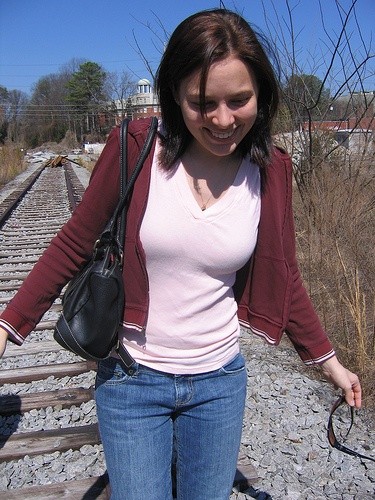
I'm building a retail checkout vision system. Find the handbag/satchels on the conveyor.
[52,115,159,367]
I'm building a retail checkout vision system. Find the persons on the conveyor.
[0,8,363,500]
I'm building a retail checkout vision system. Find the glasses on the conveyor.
[327,395,375,462]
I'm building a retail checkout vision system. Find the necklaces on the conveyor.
[187,144,233,212]
